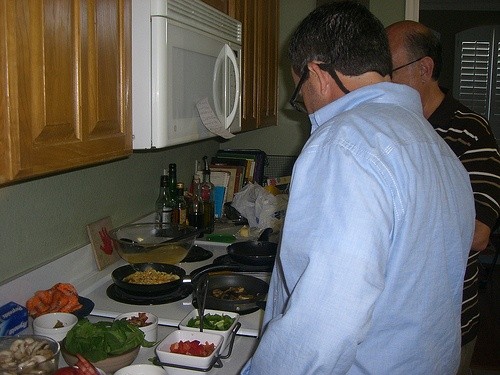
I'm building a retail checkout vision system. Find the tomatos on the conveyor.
[169,340,208,357]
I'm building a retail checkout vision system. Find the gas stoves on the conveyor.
[75,242,272,337]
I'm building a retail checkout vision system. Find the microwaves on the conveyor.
[131,0,243,151]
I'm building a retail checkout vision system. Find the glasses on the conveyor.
[288,62,329,114]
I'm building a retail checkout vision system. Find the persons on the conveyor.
[385,20,500,375]
[239,2,476,375]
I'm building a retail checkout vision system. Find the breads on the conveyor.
[240,226,250,237]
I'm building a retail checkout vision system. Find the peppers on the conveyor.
[187,313,234,331]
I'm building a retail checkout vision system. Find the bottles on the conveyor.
[154,162,214,238]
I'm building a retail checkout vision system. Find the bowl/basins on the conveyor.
[70,296,95,319]
[0,334,61,375]
[156,328,224,375]
[32,312,79,342]
[61,336,140,375]
[108,222,197,265]
[178,308,240,355]
[114,312,158,343]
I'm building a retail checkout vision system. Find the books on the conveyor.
[197,148,265,221]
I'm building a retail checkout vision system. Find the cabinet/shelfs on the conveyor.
[0,0,133,188]
[235,0,278,131]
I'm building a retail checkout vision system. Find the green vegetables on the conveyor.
[62,317,158,362]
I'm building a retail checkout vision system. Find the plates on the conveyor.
[113,364,206,375]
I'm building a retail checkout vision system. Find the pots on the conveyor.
[193,274,269,313]
[111,262,192,297]
[227,227,278,265]
